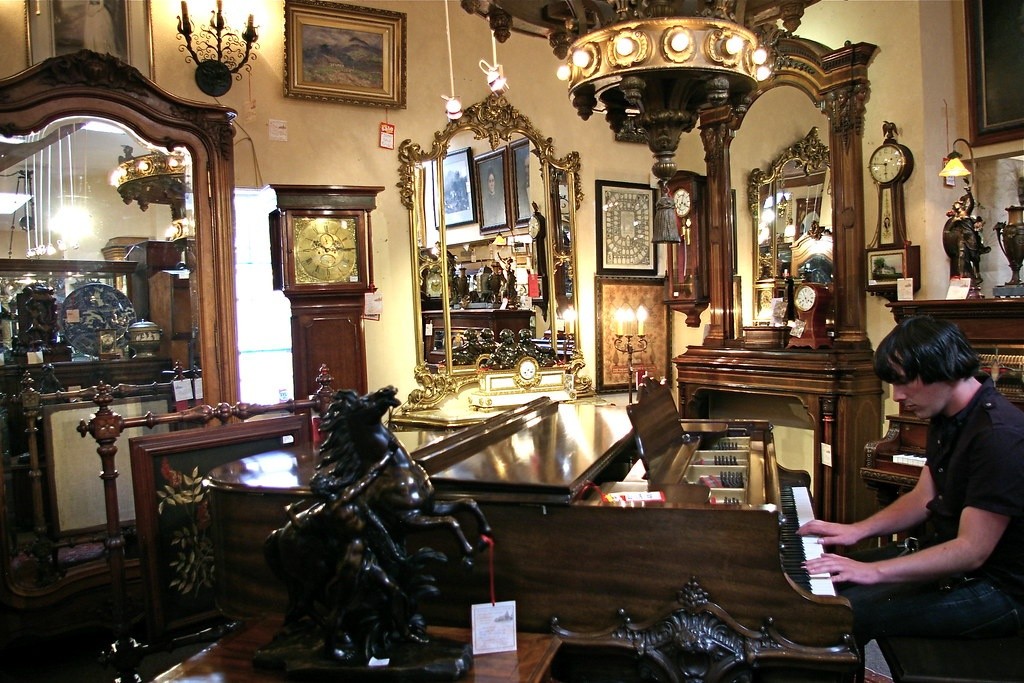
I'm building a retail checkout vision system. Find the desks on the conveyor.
[149,613,562,683]
[423,307,538,364]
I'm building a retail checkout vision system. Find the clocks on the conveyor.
[657,170,711,328]
[785,282,833,350]
[260,184,384,447]
[753,285,777,324]
[864,120,921,302]
[528,201,549,323]
[419,244,456,313]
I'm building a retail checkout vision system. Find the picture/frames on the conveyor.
[23,0,157,86]
[432,146,478,230]
[594,274,673,395]
[509,138,544,229]
[474,146,513,237]
[283,0,407,110]
[964,0,1024,149]
[595,179,658,277]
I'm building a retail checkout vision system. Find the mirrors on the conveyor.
[747,126,837,329]
[697,27,877,350]
[0,48,240,610]
[396,89,592,395]
[788,219,834,288]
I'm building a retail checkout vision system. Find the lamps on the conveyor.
[176,0,260,98]
[489,229,519,311]
[444,0,827,244]
[109,140,194,245]
[938,139,991,299]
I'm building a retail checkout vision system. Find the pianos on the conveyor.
[202,374,863,683]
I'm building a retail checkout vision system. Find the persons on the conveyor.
[488,328,517,370]
[517,328,561,367]
[482,168,503,224]
[320,533,405,662]
[464,329,498,355]
[283,436,407,568]
[793,313,1024,680]
[496,251,516,298]
[948,176,982,281]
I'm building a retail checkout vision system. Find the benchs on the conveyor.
[876,630,1023,683]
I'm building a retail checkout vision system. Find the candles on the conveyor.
[614,304,647,337]
